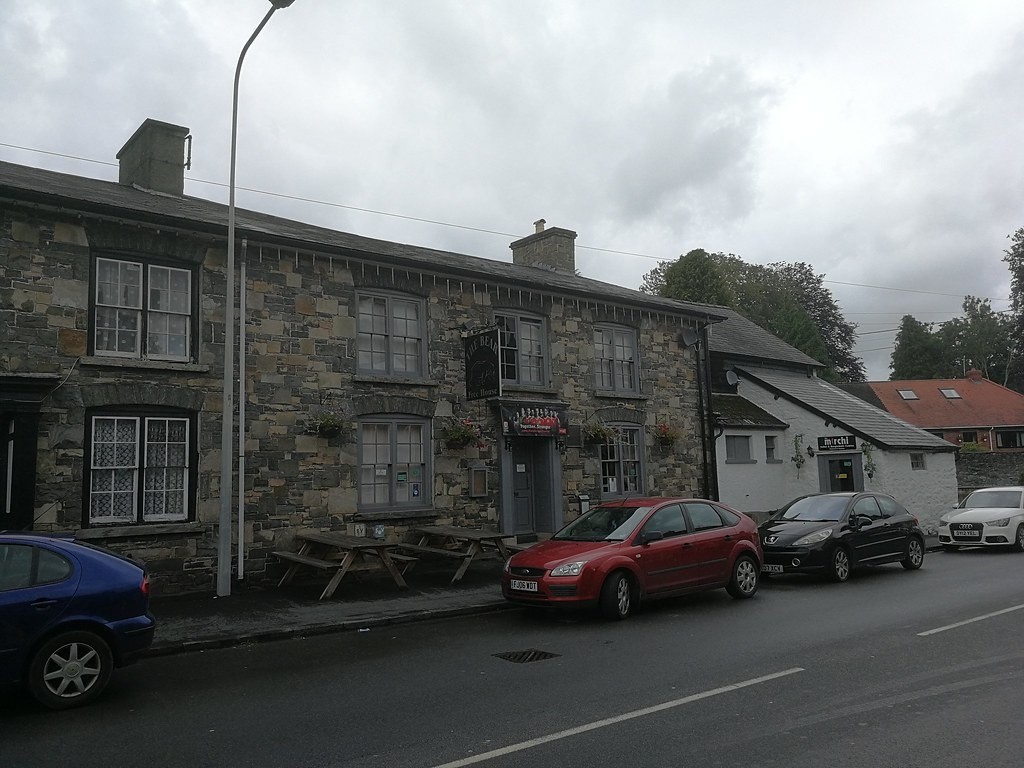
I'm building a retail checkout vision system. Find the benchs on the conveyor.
[271,549,344,572]
[458,538,529,553]
[344,546,418,565]
[398,542,472,560]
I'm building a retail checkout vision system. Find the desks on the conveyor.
[295,532,409,600]
[404,524,513,583]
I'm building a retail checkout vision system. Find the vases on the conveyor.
[446,435,473,450]
[658,436,674,448]
[590,434,610,446]
[317,422,346,438]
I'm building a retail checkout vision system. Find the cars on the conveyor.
[500,497,762,624]
[756,491,924,582]
[938,486,1023,551]
[1,529,152,717]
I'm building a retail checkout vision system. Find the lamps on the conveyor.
[806,446,815,458]
[503,435,512,451]
[556,433,567,449]
[860,443,868,455]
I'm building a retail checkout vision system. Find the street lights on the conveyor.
[215,1,295,596]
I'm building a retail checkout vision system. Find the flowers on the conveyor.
[434,416,493,453]
[651,424,682,441]
[578,419,626,445]
[298,391,359,426]
